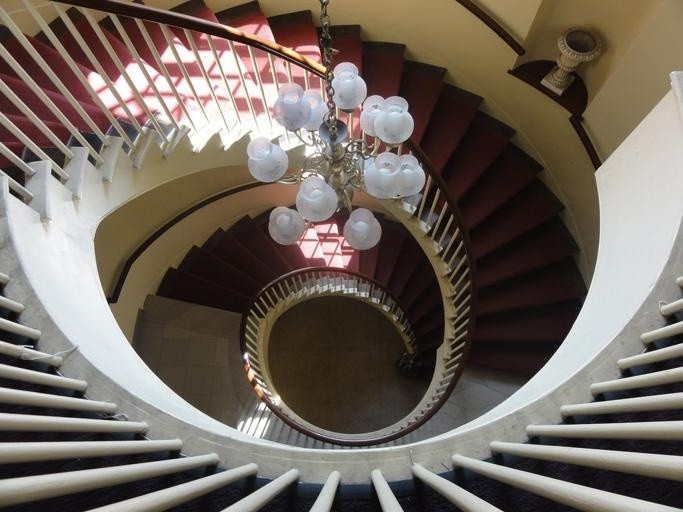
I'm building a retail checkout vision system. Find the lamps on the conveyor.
[241,1,428,253]
